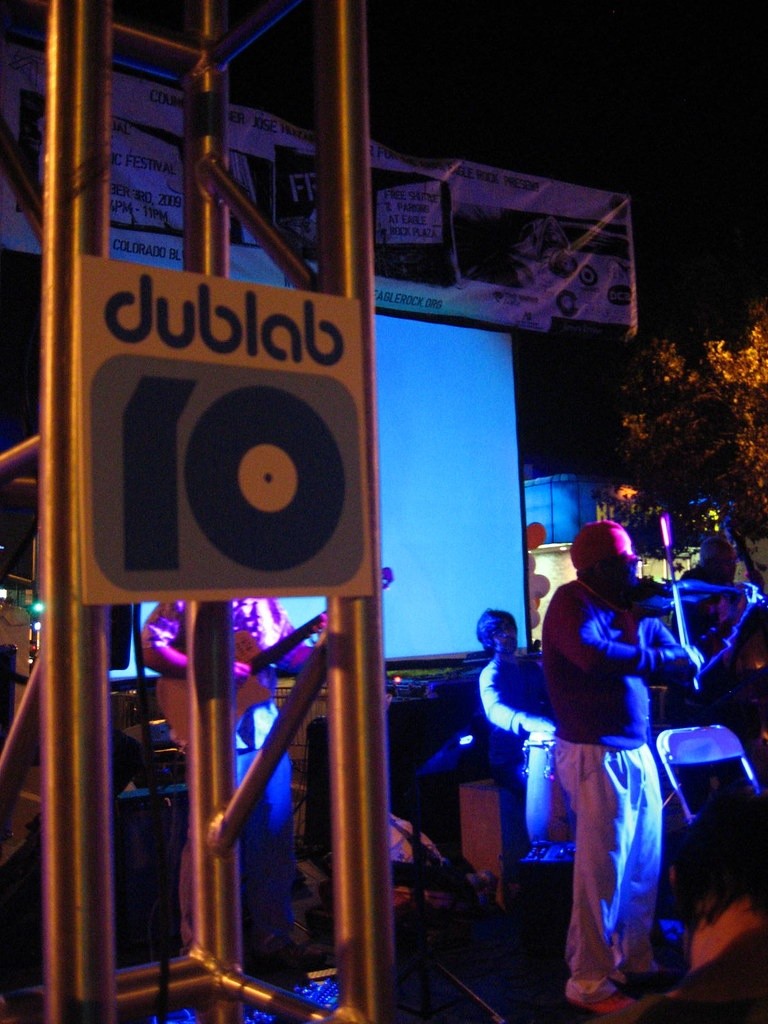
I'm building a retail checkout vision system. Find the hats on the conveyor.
[569,519,642,575]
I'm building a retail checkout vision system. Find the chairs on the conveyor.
[657,723,763,826]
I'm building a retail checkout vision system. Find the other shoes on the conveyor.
[610,960,675,992]
[564,977,638,1016]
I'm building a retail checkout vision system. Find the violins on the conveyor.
[628,576,768,615]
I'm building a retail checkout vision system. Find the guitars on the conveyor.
[154,566,395,744]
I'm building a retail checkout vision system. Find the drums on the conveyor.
[519,727,576,850]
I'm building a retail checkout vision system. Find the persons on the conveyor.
[541,520,768,1014]
[138,596,331,968]
[580,786,767,1024]
[473,608,549,795]
